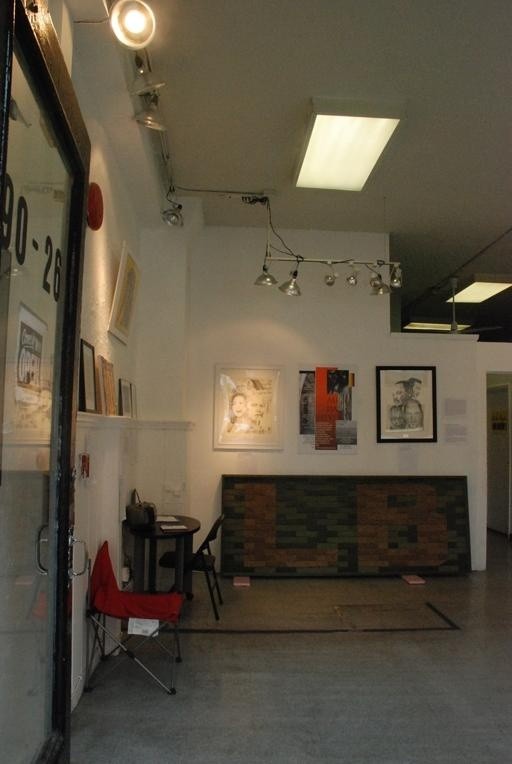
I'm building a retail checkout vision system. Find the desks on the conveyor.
[121,514,200,601]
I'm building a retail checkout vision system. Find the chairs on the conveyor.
[158,511,226,621]
[83,539,187,695]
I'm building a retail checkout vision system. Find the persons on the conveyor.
[224,378,268,433]
[390,377,424,430]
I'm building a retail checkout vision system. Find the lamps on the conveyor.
[295,112,400,192]
[254,245,304,296]
[322,259,403,296]
[161,186,183,230]
[111,2,169,138]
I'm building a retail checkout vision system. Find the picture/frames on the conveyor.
[375,364,438,443]
[79,237,139,420]
[213,363,284,452]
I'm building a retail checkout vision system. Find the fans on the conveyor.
[445,276,504,334]
